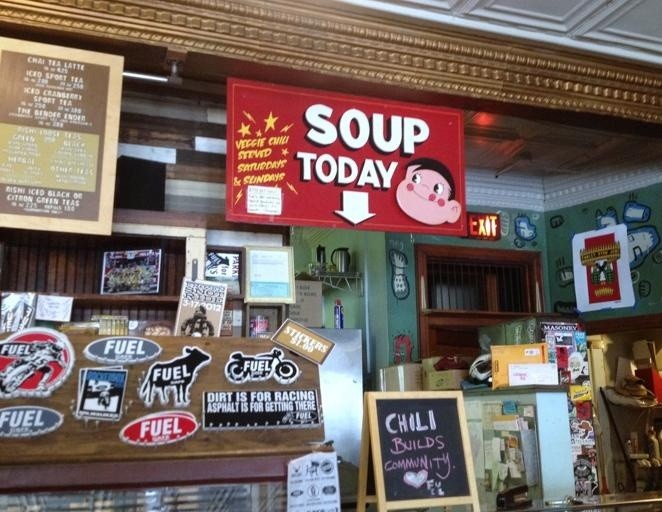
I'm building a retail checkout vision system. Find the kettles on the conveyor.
[330,246,351,273]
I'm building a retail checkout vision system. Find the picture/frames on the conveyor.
[244,244,296,304]
[204,245,246,300]
[99,245,163,295]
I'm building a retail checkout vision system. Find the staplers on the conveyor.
[496,485,533,512]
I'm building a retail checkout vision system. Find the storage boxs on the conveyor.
[421,355,473,392]
[428,369,468,392]
[478,317,583,373]
[378,363,423,393]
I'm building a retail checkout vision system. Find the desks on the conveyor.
[495,492,662,512]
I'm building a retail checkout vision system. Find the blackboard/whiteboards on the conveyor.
[367,390,477,510]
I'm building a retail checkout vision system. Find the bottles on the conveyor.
[333,299,344,329]
[647,426,661,458]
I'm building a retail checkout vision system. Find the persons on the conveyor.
[103,261,153,293]
[396,157,462,227]
[0,340,66,392]
